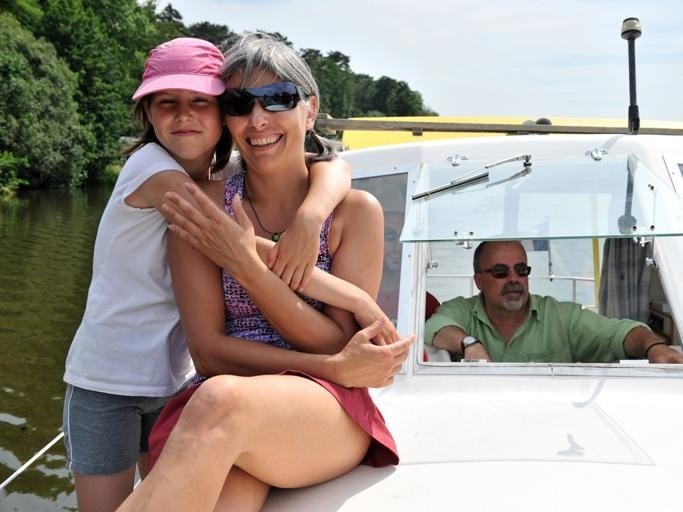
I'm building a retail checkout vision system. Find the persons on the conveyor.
[376,210,456,362]
[112,34,417,512]
[425,241,683,364]
[61,37,402,512]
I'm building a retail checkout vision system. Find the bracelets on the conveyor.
[644,342,669,358]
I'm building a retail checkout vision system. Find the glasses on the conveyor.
[476,263,531,278]
[221,81,308,116]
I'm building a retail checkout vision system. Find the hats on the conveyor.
[131,37,227,101]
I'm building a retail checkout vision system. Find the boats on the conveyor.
[0,16,682,510]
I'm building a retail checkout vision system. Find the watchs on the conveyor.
[461,336,484,354]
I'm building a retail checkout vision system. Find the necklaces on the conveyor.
[244,173,287,242]
